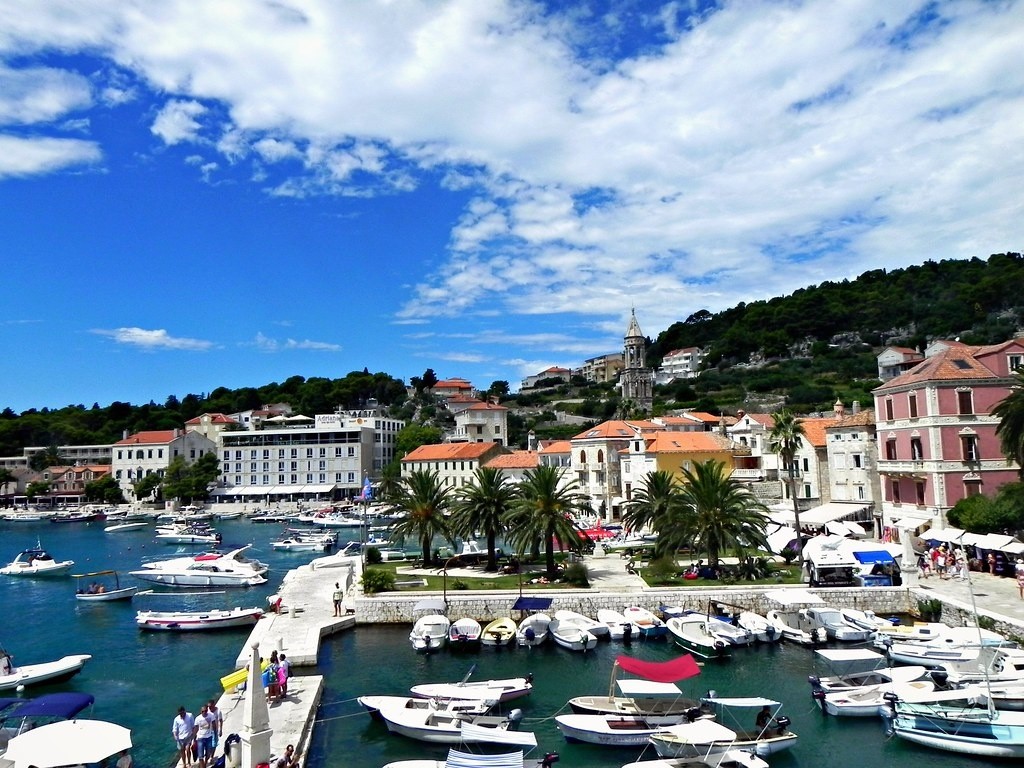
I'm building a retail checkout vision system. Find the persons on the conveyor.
[1015,559,1024,599]
[254,507,261,512]
[28,554,34,567]
[988,552,995,574]
[917,545,966,579]
[277,744,299,768]
[89,582,104,593]
[677,559,718,580]
[333,582,343,616]
[266,596,282,614]
[173,700,223,768]
[268,650,292,703]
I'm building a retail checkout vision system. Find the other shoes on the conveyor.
[333,614,336,617]
[280,695,286,698]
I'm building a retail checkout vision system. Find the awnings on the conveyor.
[893,516,929,532]
[300,483,336,493]
[269,484,304,494]
[210,486,246,494]
[786,502,873,534]
[239,485,274,494]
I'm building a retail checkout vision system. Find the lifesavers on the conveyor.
[799,613,804,619]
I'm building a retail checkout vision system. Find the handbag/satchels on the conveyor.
[288,666,293,676]
[191,740,198,762]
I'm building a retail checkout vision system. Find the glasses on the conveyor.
[202,710,208,712]
[289,750,294,752]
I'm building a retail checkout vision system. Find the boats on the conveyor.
[647,696,799,758]
[621,747,770,768]
[409,588,1024,710]
[0,500,659,587]
[380,706,523,744]
[808,666,926,695]
[410,662,534,706]
[380,748,560,768]
[880,533,1024,759]
[812,679,935,717]
[134,606,265,630]
[75,586,137,602]
[356,694,498,723]
[0,535,74,577]
[568,653,703,716]
[554,710,717,747]
[0,639,92,690]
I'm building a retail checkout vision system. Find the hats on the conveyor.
[964,549,967,551]
[266,595,269,602]
[924,551,929,554]
[1017,559,1023,563]
[941,543,946,546]
